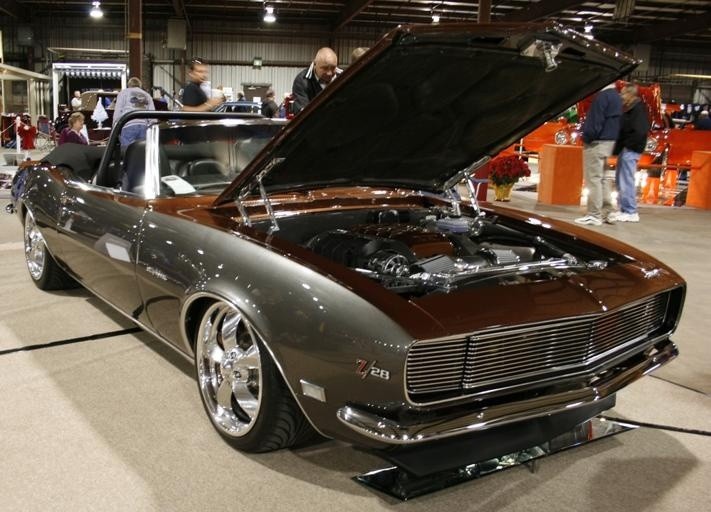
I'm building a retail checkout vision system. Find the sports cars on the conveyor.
[9,18,687,483]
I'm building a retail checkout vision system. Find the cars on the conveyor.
[52,91,169,139]
[554,77,676,165]
[279,92,294,119]
[213,101,289,120]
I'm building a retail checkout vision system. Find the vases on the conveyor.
[492,184,515,202]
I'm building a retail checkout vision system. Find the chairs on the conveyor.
[122,138,169,194]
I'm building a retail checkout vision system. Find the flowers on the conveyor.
[489,155,532,186]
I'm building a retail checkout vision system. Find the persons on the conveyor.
[60,112,107,146]
[574,82,622,228]
[112,77,159,149]
[181,46,371,127]
[71,90,83,111]
[611,85,648,222]
[693,110,711,130]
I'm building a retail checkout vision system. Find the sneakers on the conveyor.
[575,204,641,226]
